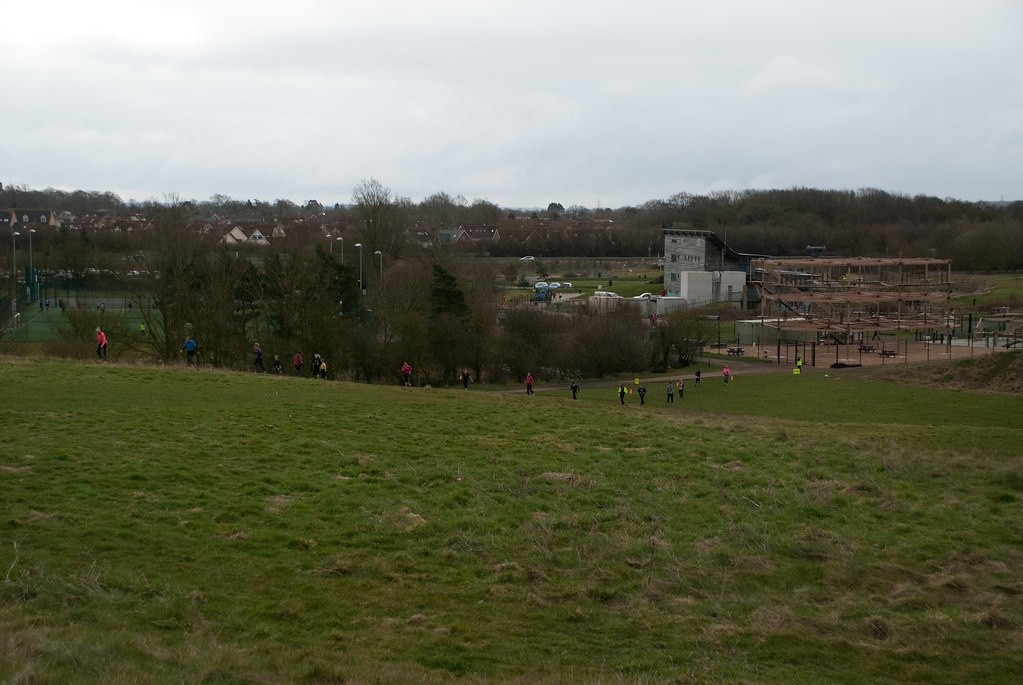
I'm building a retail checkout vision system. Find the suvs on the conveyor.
[519,256,536,264]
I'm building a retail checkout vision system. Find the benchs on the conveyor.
[858,348,867,350]
[732,350,744,353]
[878,352,889,355]
[727,350,737,352]
[887,353,897,356]
[867,349,876,350]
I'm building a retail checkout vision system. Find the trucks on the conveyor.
[594,291,621,298]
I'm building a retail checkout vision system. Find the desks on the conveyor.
[727,347,744,355]
[878,349,896,358]
[859,345,875,353]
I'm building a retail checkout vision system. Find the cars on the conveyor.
[549,282,561,289]
[562,283,573,289]
[532,281,548,290]
[0,267,162,286]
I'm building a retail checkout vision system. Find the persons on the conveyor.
[617,385,628,405]
[678,379,684,398]
[58,296,65,317]
[294,351,303,377]
[665,381,674,403]
[695,369,700,386]
[570,381,580,400]
[39,300,43,315]
[45,301,50,312]
[312,354,322,380]
[524,373,535,396]
[721,365,731,385]
[401,361,411,387]
[95,327,107,363]
[459,368,474,390]
[319,359,328,381]
[272,355,281,374]
[179,336,200,368]
[139,322,146,335]
[797,355,802,372]
[638,385,646,405]
[95,302,105,313]
[253,342,267,374]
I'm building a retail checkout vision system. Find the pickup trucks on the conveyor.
[633,292,662,299]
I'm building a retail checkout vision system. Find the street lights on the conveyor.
[374,250,384,289]
[354,243,362,291]
[336,237,344,264]
[28,229,38,281]
[326,234,332,254]
[11,231,21,279]
